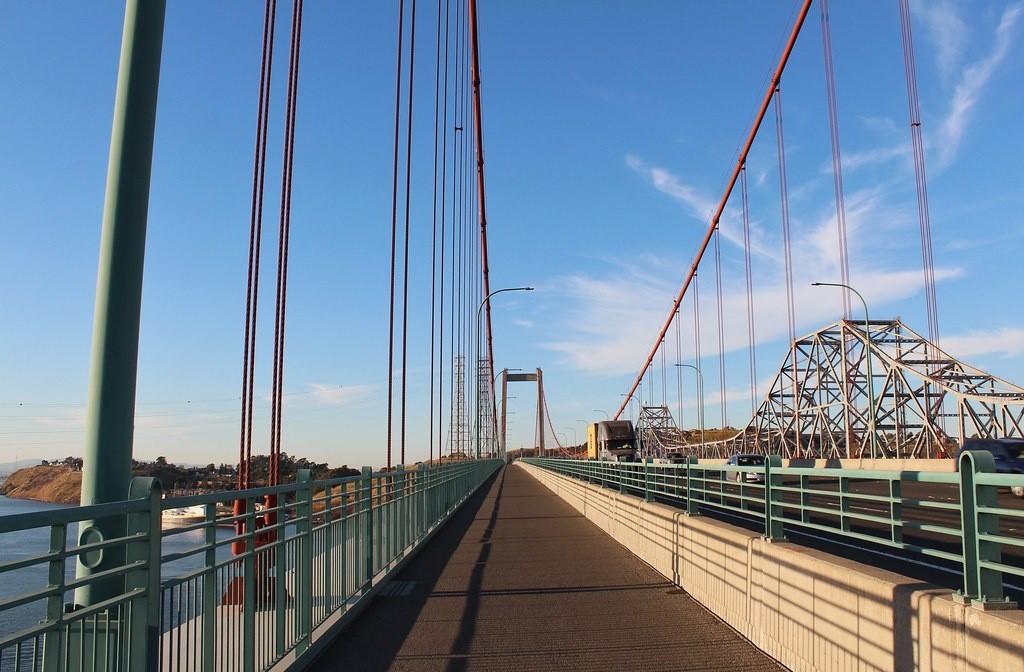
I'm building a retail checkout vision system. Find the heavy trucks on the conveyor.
[585,420,638,463]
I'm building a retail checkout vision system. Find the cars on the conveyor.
[724,454,765,484]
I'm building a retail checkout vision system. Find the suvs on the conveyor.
[955,438,1024,498]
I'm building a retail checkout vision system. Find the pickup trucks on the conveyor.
[659,453,684,469]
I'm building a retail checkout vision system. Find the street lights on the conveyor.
[811,282,878,458]
[577,419,589,426]
[673,363,705,459]
[475,286,535,460]
[547,426,577,460]
[593,409,609,421]
[620,393,642,411]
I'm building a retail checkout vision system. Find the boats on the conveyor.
[159,504,269,530]
